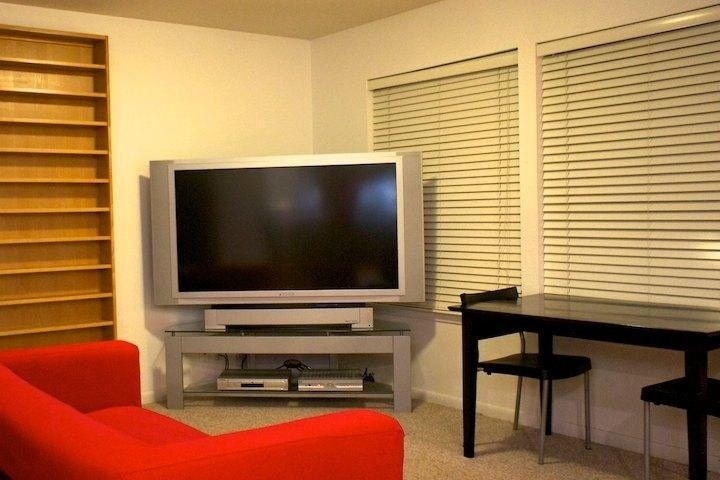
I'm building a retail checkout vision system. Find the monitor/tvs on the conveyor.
[149,150,425,306]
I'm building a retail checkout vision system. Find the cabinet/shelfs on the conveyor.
[0,23,116,348]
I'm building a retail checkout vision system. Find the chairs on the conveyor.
[460,286,592,465]
[640,376,720,480]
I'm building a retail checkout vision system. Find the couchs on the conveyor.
[0,341,404,480]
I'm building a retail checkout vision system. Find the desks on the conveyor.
[163,319,412,413]
[447,292,720,480]
